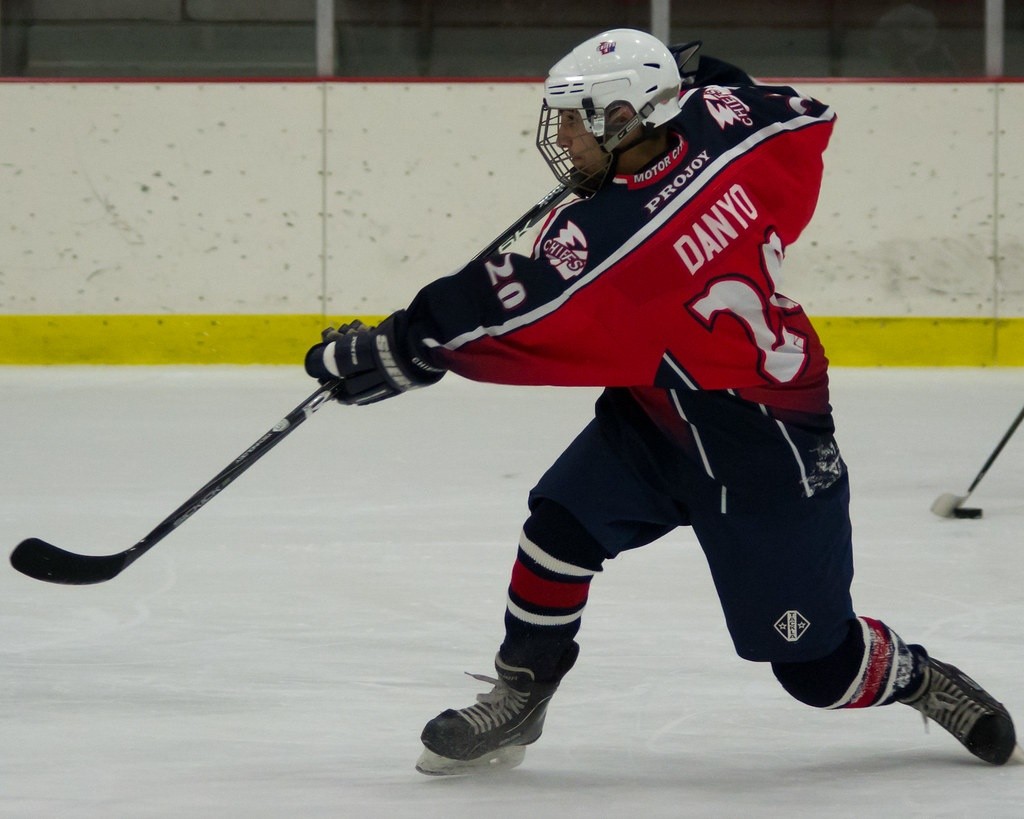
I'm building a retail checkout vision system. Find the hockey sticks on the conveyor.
[8,166,581,588]
[928,408,1024,520]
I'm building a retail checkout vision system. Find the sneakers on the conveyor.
[415,641,580,776]
[900,645,1024,766]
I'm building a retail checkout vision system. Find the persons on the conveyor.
[305,27,1023,775]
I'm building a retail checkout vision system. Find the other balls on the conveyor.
[952,507,983,520]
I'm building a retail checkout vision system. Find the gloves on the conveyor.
[305,308,447,407]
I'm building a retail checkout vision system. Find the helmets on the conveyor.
[536,29,683,199]
